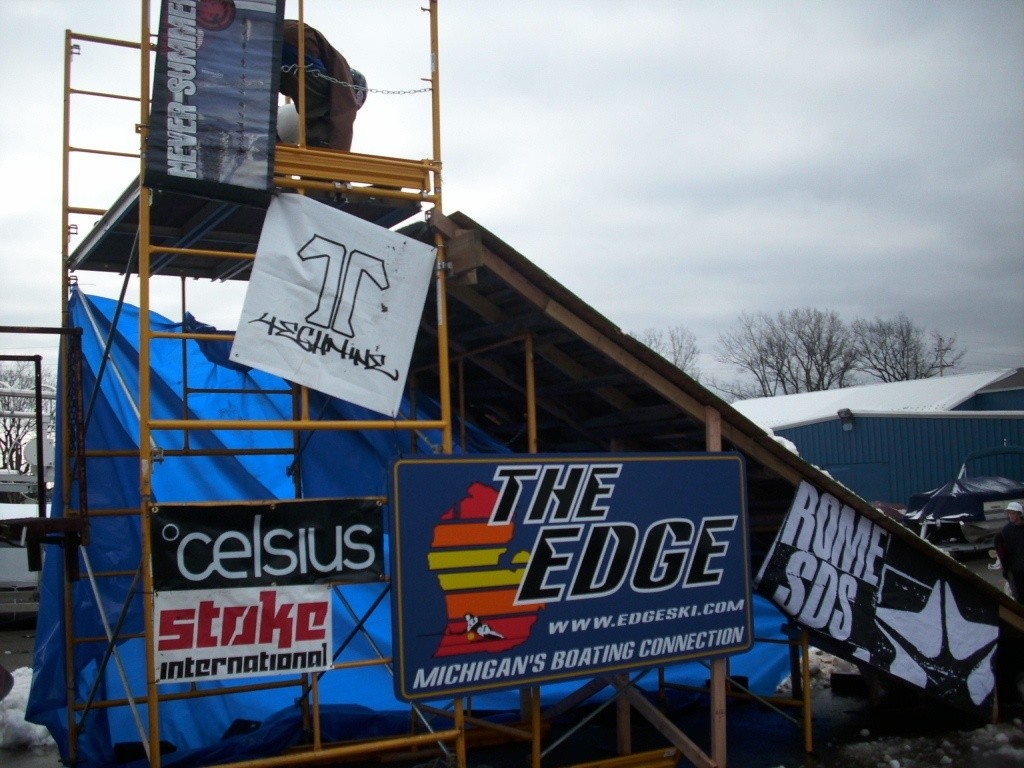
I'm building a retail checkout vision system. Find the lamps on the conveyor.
[837,408,855,431]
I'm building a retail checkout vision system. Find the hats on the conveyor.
[1005,502,1023,513]
[350,68,369,111]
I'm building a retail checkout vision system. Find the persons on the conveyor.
[996,502,1024,609]
[280,19,358,196]
